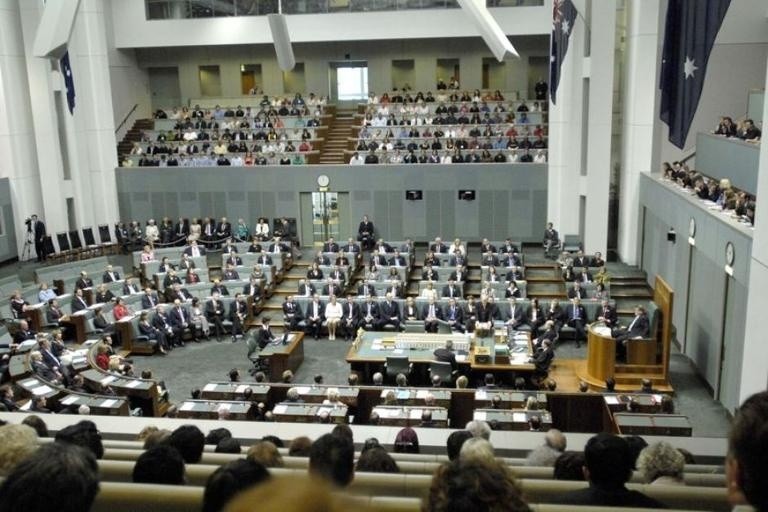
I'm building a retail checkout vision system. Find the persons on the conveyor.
[119,76,549,169]
[663,115,761,224]
[0,215,767,511]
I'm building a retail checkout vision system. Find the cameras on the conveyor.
[24,217,32,225]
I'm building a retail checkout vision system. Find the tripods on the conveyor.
[20,225,35,262]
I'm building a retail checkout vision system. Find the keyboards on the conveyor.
[272,340,282,345]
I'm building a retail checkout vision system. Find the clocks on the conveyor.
[316,174,330,187]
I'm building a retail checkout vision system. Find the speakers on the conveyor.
[668,230,675,243]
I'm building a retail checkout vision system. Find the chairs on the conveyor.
[543,230,560,250]
[563,235,582,253]
[45,217,300,266]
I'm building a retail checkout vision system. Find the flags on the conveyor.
[550,1,577,104]
[660,1,730,150]
[60,51,75,114]
